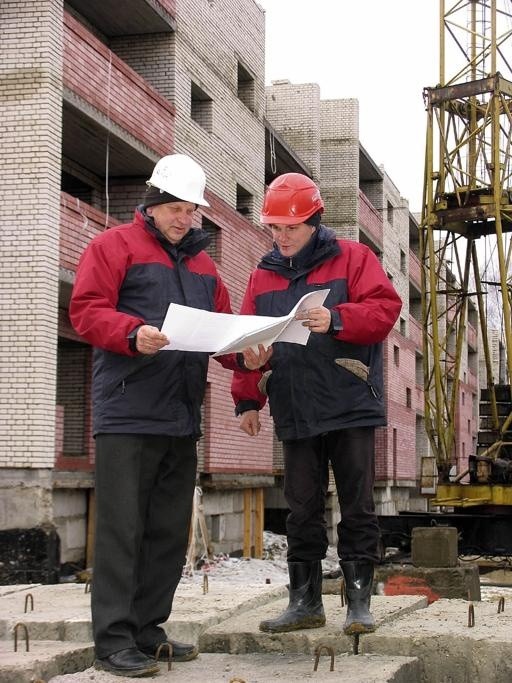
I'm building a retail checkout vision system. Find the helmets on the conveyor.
[258,172,324,225]
[145,154,210,208]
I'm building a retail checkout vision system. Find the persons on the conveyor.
[227,168,404,640]
[66,151,277,679]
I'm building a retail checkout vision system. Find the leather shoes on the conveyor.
[93,646,160,677]
[141,634,199,662]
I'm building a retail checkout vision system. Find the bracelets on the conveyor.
[243,358,251,370]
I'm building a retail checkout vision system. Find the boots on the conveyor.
[338,559,377,635]
[258,560,326,632]
